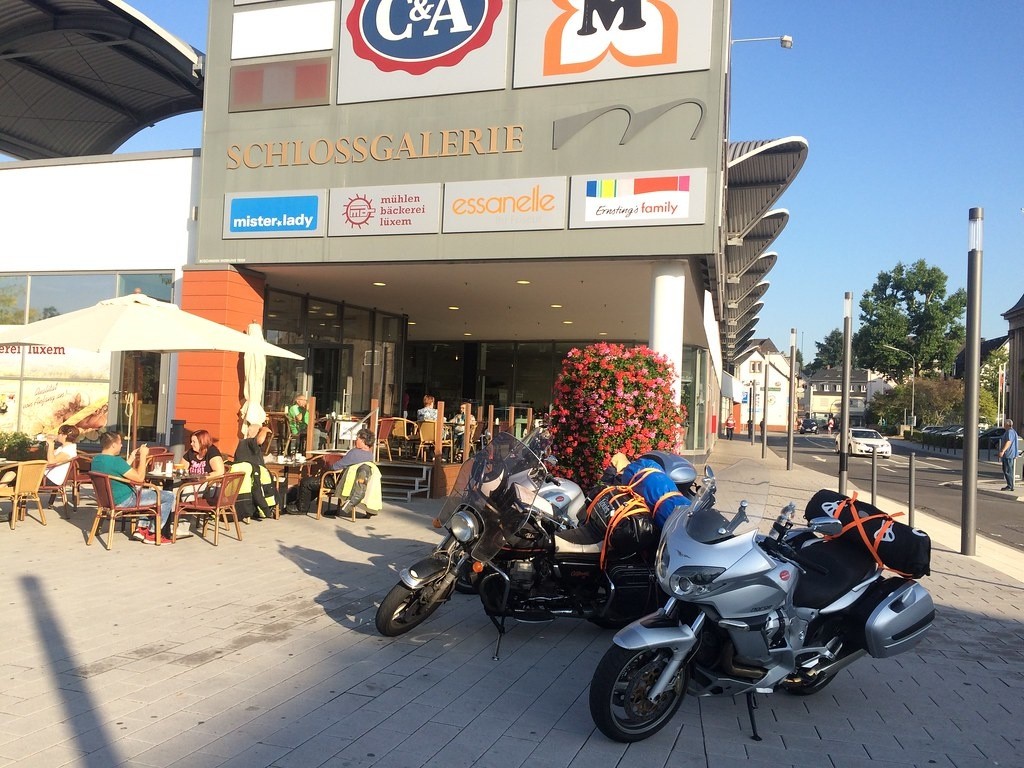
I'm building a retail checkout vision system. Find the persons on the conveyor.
[0,424,80,487]
[828,418,834,428]
[417,395,449,461]
[234,425,272,467]
[760,418,764,435]
[170,430,224,535]
[449,402,475,461]
[725,413,736,440]
[287,395,321,450]
[284,429,375,515]
[90,432,174,545]
[998,419,1018,490]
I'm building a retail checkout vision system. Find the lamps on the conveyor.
[731,34,794,49]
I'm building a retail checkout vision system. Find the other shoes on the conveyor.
[0,512,20,523]
[1001,486,1014,492]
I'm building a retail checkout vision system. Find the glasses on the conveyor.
[58,432,65,435]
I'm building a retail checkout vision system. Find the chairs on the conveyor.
[0,447,279,550]
[298,454,382,522]
[265,411,489,465]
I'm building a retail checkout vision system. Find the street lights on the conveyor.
[883,345,915,436]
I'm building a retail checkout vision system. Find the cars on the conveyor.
[800,419,819,435]
[978,427,1023,449]
[834,429,892,459]
[921,425,964,439]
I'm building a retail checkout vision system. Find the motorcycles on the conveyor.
[376,423,701,661]
[588,457,935,743]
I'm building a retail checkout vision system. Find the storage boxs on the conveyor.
[855,577,935,659]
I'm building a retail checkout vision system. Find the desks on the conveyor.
[265,461,317,515]
[145,470,210,540]
[318,417,368,450]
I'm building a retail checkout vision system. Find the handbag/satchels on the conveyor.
[622,459,693,526]
[803,488,933,578]
[203,480,221,507]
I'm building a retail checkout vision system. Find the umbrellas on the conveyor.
[0,287,306,466]
[239,321,267,439]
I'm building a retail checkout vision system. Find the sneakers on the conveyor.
[143,532,172,545]
[287,503,307,515]
[133,527,151,540]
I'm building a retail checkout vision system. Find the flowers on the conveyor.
[523,342,690,496]
